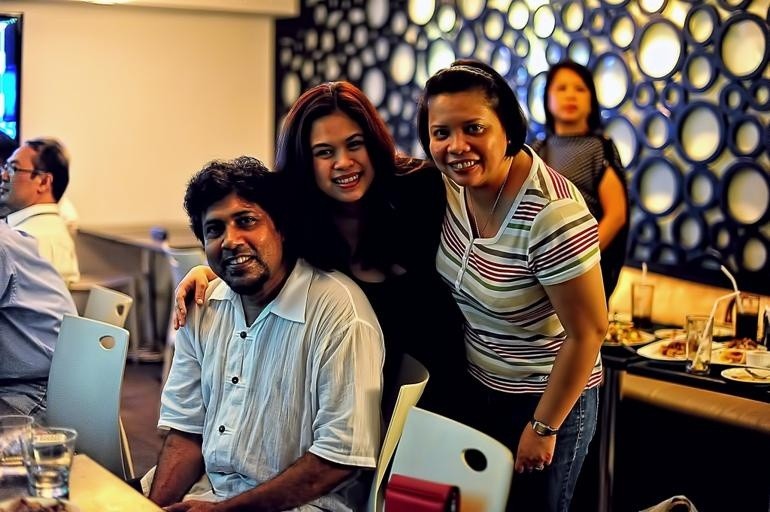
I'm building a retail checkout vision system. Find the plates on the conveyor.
[1,496,81,512]
[653,328,686,339]
[600,329,657,347]
[721,367,770,384]
[635,339,690,361]
[705,348,770,369]
[719,341,767,350]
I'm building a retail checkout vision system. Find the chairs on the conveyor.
[382,406,514,512]
[367,352,431,512]
[75,221,205,364]
[161,239,204,390]
[70,276,139,363]
[82,285,134,479]
[46,312,131,483]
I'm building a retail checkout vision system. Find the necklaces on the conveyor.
[465,166,509,239]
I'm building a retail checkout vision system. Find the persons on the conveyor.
[415,59,610,512]
[0,217,80,431]
[1,137,81,296]
[173,80,460,512]
[123,155,387,512]
[524,60,630,311]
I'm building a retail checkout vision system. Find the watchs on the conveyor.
[530,415,559,437]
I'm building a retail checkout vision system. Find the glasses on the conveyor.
[1,163,33,176]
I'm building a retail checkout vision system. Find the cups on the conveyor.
[685,313,714,375]
[631,283,654,330]
[19,426,79,501]
[744,350,769,367]
[0,413,35,467]
[735,293,760,343]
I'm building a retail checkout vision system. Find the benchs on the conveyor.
[608,259,770,432]
